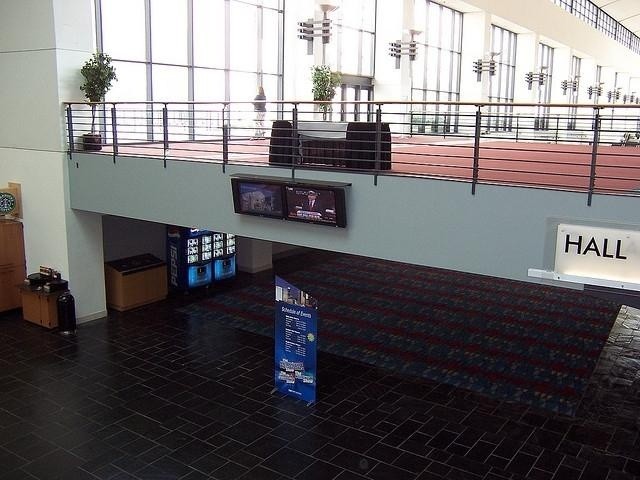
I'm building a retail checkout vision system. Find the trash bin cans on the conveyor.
[56,290,76,336]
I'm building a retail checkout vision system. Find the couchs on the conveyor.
[268,120,392,170]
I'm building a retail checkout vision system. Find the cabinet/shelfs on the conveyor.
[104,253,168,313]
[0,219,27,312]
[15,288,76,330]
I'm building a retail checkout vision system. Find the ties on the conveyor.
[309,200,313,211]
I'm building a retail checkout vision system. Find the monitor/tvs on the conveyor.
[231,178,347,228]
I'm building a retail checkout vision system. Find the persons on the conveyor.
[251,87,268,139]
[302,190,323,215]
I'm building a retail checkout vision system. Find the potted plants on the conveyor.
[79,46,119,150]
